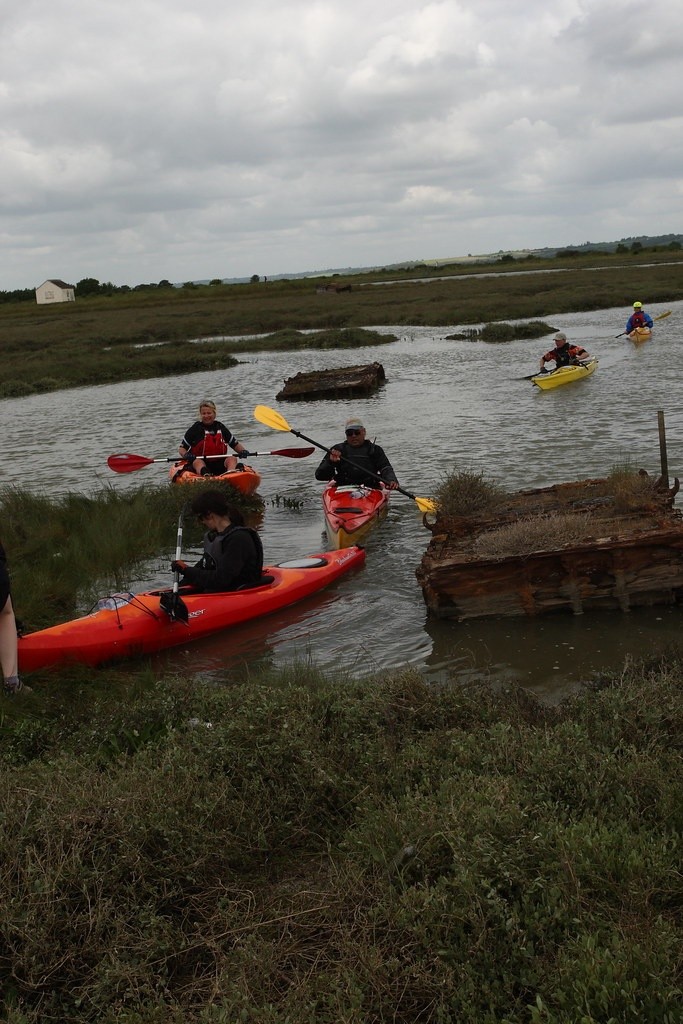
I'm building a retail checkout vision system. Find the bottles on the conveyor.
[98,593,124,610]
[107,593,135,611]
[538,374,549,377]
[351,490,368,499]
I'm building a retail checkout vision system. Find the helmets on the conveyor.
[633,302,642,311]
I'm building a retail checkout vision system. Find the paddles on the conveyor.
[521,361,575,379]
[254,405,443,512]
[159,500,190,627]
[108,447,315,473]
[615,311,671,338]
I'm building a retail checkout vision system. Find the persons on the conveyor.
[171,489,255,594]
[540,332,589,374]
[624,301,653,335]
[179,400,248,477]
[0,542,35,697]
[315,417,399,491]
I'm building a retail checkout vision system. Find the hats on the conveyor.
[345,418,363,432]
[553,333,566,340]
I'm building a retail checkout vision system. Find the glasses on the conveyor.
[199,512,213,522]
[346,430,361,436]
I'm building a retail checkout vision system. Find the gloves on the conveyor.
[171,560,187,574]
[239,450,249,459]
[569,359,579,365]
[540,367,548,374]
[184,453,196,462]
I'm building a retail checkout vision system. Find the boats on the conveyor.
[170,461,261,494]
[18,544,366,674]
[531,356,599,389]
[323,480,389,551]
[629,327,652,342]
